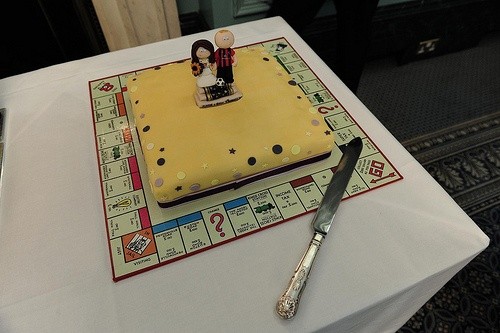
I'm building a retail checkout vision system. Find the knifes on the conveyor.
[276,135,366,320]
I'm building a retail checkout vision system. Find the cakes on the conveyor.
[126,30,335,210]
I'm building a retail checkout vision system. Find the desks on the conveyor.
[0,13,490,332]
